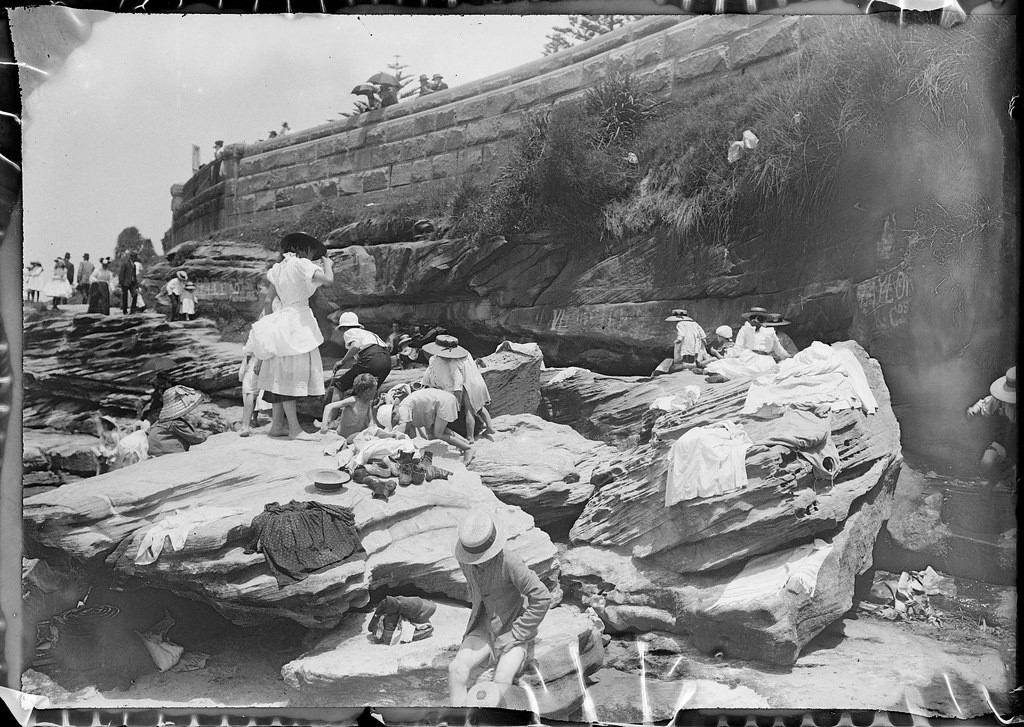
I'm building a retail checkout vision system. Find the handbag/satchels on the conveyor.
[367,594,436,645]
[136,293,146,308]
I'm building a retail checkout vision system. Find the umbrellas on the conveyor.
[351,84,377,95]
[366,73,404,88]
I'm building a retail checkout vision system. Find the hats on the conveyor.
[421,335,468,359]
[279,232,327,261]
[664,309,694,322]
[157,385,203,424]
[741,308,767,321]
[334,311,364,332]
[31,251,110,267]
[376,399,398,433]
[306,468,352,491]
[990,366,1016,404]
[755,312,791,326]
[185,281,195,290]
[715,324,734,339]
[451,510,508,564]
[398,334,411,347]
[177,270,188,281]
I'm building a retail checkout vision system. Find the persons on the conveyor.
[24,250,144,315]
[418,72,448,97]
[664,306,791,374]
[965,364,1016,491]
[268,121,290,138]
[367,84,399,108]
[314,310,496,466]
[237,230,335,442]
[146,385,208,456]
[165,269,200,321]
[447,515,551,708]
[89,256,113,316]
[212,139,225,158]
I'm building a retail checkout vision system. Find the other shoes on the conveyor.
[696,360,706,368]
[691,367,705,374]
[705,375,723,382]
[350,448,452,503]
